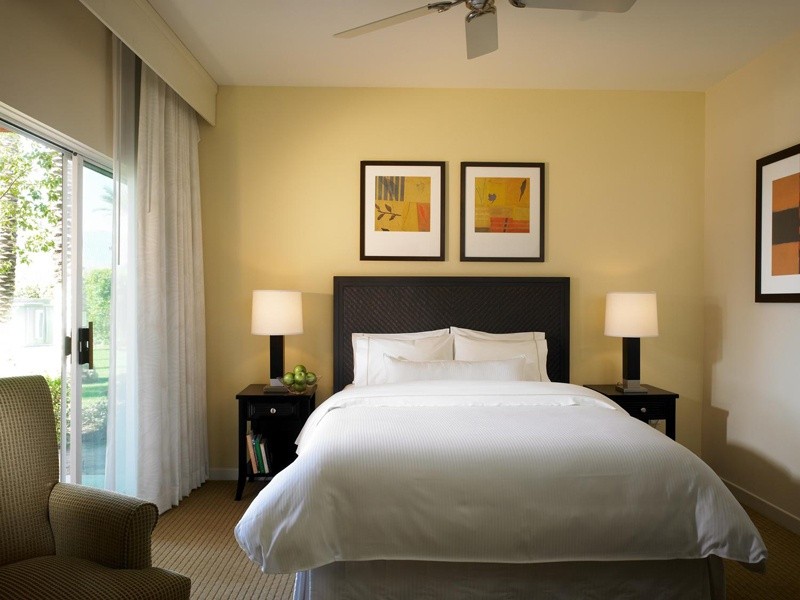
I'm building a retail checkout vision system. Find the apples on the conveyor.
[284,364,317,392]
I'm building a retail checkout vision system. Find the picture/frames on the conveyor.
[460,161,544,262]
[755,143,800,303]
[360,160,445,261]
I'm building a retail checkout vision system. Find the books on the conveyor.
[246,431,270,473]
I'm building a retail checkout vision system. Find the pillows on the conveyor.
[451,325,553,382]
[451,354,526,383]
[373,352,456,385]
[353,327,454,384]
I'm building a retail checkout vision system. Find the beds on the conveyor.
[233,276,769,600]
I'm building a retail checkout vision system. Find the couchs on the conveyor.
[0,374,191,600]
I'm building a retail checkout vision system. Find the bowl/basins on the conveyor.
[279,377,320,395]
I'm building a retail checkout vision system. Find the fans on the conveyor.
[334,0,637,59]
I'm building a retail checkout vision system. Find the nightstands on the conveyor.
[582,383,680,441]
[234,384,318,502]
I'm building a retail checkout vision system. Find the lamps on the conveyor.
[604,292,658,394]
[251,289,303,391]
